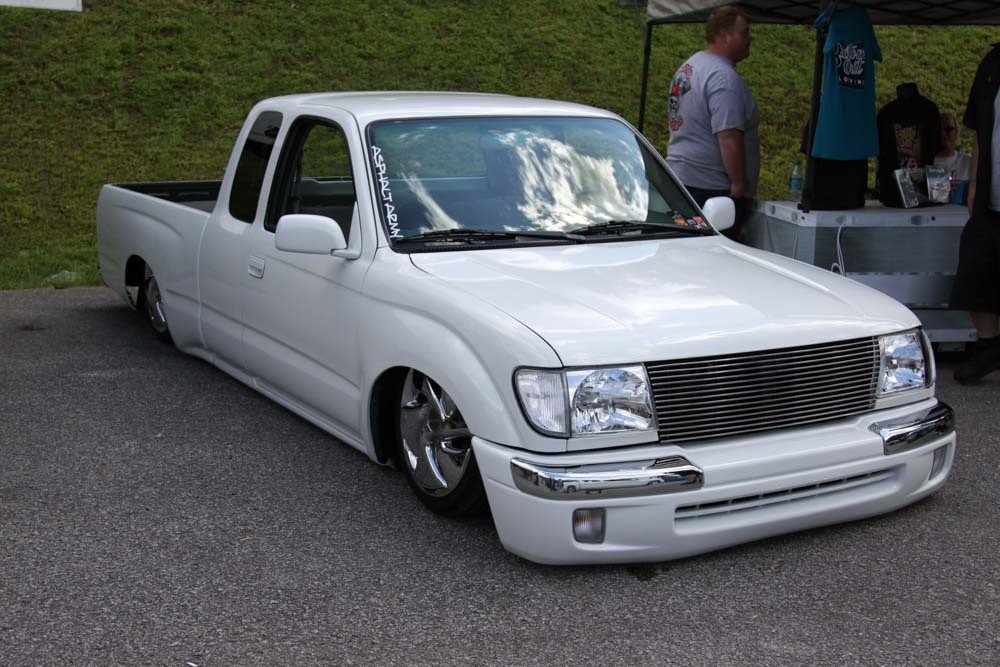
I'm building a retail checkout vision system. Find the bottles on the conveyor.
[788,159,803,202]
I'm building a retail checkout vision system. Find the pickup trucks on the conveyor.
[93,88,958,566]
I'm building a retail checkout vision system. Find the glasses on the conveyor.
[941,125,960,132]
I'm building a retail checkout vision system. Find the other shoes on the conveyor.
[954,336,1000,382]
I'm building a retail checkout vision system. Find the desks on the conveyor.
[749,200,977,353]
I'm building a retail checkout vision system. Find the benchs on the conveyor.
[295,178,543,232]
